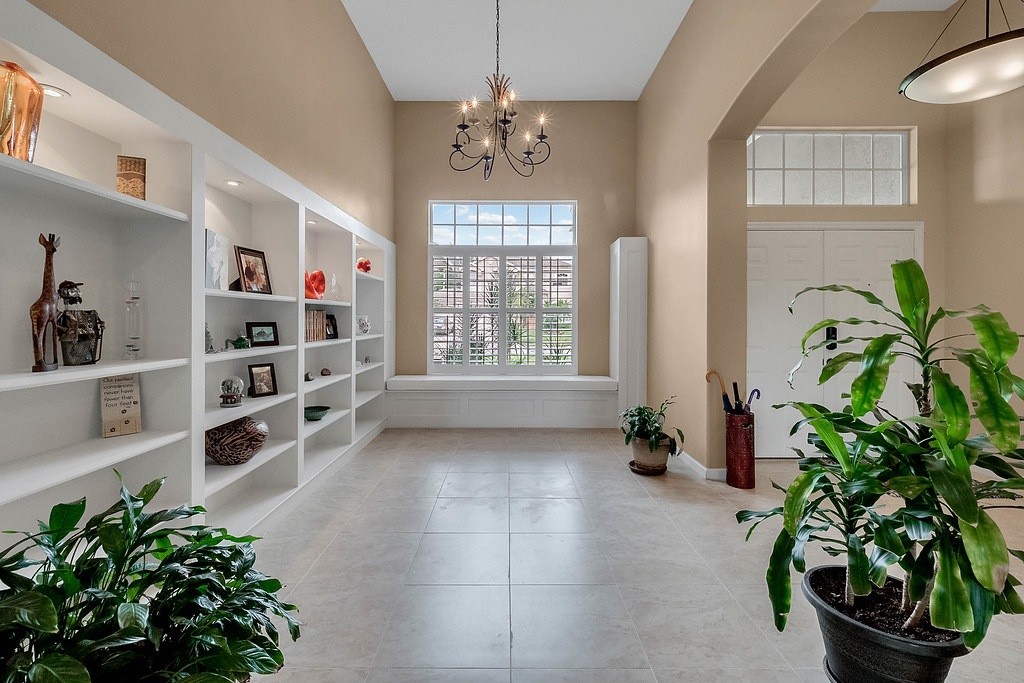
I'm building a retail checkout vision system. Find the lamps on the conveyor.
[449,0,551,182]
[897,0,1024,105]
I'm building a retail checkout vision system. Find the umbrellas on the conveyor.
[705,370,761,415]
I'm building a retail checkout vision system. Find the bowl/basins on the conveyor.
[303,406,332,421]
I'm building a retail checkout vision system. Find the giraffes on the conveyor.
[30,232,60,366]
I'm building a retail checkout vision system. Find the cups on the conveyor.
[0,60,44,163]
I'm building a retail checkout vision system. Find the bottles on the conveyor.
[126,281,145,360]
[356,315,372,336]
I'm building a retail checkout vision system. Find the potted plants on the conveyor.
[733,258,1024,683]
[618,394,685,477]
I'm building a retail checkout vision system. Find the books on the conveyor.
[305,310,326,342]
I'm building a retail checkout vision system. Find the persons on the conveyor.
[245,263,257,282]
[257,372,269,385]
[256,328,266,336]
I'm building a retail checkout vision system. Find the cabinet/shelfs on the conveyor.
[0,0,398,662]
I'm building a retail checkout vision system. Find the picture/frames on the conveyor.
[245,321,280,347]
[248,362,279,398]
[234,245,272,295]
[326,314,338,339]
